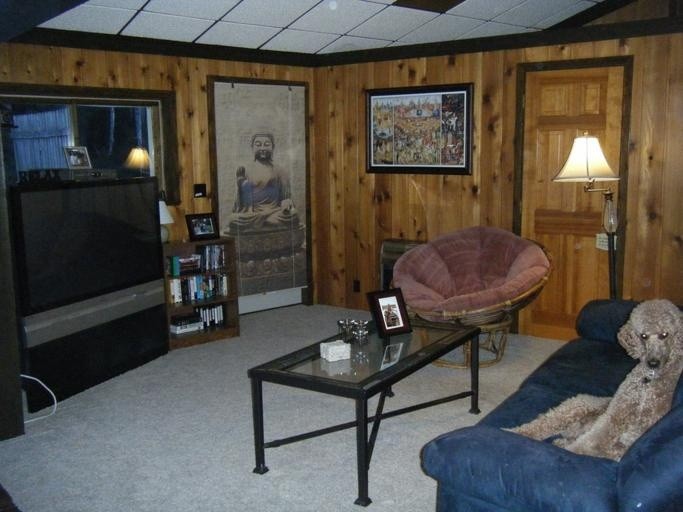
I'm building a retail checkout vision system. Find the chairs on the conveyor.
[392,226,552,367]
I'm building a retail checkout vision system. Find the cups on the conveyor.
[337,318,368,347]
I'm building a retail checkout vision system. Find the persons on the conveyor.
[384,301,399,328]
[383,343,399,365]
[227,128,298,236]
[372,137,464,166]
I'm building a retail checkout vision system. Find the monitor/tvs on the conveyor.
[7,177,169,348]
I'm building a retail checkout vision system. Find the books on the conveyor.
[165,240,230,335]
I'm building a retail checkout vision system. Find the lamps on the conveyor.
[124,147,152,177]
[551,131,621,301]
[159,200,175,243]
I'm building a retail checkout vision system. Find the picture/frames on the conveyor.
[206,73,314,291]
[63,146,93,170]
[186,214,217,239]
[365,287,412,338]
[365,82,474,176]
[373,335,413,374]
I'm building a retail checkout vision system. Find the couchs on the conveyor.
[419,298,683,512]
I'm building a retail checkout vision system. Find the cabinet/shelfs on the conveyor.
[164,239,241,347]
